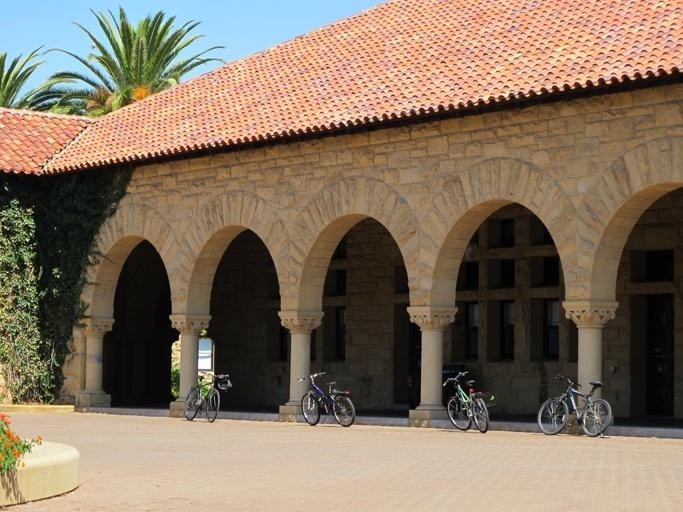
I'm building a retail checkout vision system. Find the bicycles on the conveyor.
[184,372,229,422]
[298,372,355,427]
[443,371,489,433]
[538,374,612,437]
